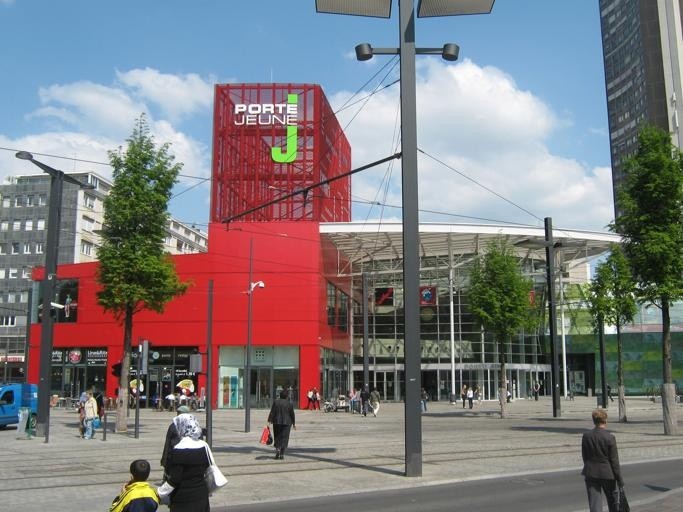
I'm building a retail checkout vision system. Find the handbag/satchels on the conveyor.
[620,488,630,511]
[93,419,101,428]
[259,428,273,446]
[204,464,228,493]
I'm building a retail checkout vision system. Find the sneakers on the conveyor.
[276,450,283,459]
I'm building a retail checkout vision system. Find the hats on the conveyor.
[177,406,189,413]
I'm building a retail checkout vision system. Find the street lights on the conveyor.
[589,282,611,410]
[314,0,494,477]
[14,150,96,440]
[512,216,585,417]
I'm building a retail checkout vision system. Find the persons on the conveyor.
[307,386,381,418]
[109,459,160,512]
[459,383,511,409]
[420,387,428,412]
[533,380,541,393]
[606,382,614,402]
[266,387,297,460]
[154,412,214,512]
[581,407,624,512]
[72,384,207,439]
[527,388,532,399]
[160,404,193,485]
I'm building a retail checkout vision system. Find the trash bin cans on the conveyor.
[16,407,32,440]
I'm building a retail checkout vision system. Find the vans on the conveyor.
[0,383,38,430]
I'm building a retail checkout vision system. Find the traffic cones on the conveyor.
[259,428,268,445]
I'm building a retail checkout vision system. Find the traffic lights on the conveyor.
[142,341,152,374]
